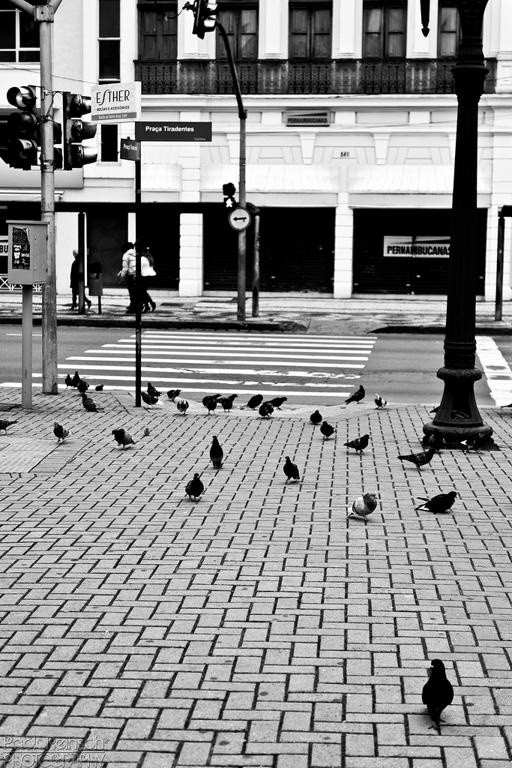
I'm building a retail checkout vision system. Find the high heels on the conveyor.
[142,304,151,315]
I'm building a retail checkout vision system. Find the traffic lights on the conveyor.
[193,0,218,38]
[5,82,37,169]
[225,197,235,209]
[64,91,97,173]
[223,184,235,195]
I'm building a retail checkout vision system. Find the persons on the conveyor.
[118,242,136,313]
[140,245,157,313]
[70,250,91,310]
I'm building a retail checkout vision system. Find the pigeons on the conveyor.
[310,410,336,440]
[182,471,203,503]
[111,429,135,450]
[343,433,370,454]
[347,493,378,522]
[344,384,365,405]
[283,456,300,483]
[52,421,70,444]
[421,657,454,731]
[141,380,288,419]
[373,393,388,411]
[397,443,438,470]
[1,419,18,435]
[210,435,223,470]
[415,490,458,513]
[64,371,104,411]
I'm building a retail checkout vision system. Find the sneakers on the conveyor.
[150,300,158,314]
[84,299,93,313]
[125,301,136,315]
[69,301,77,312]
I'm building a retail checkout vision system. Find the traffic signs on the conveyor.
[123,120,216,163]
